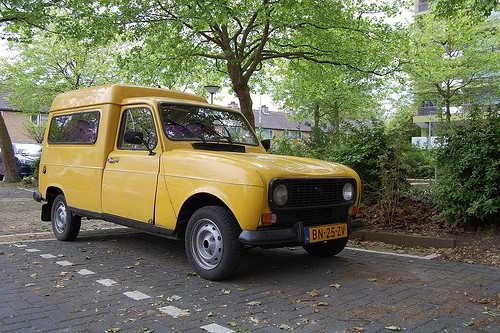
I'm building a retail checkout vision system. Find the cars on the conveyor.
[0,143,42,181]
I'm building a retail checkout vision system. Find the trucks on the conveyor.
[32,84,362,280]
